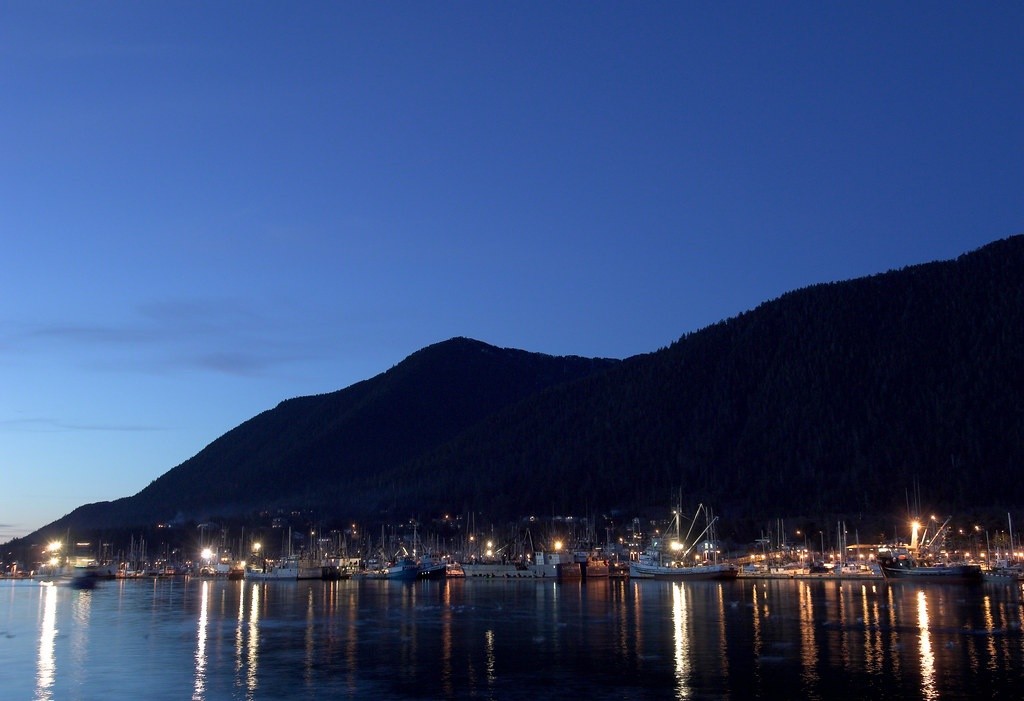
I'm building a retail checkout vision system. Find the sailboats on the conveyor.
[29,504,1023,593]
